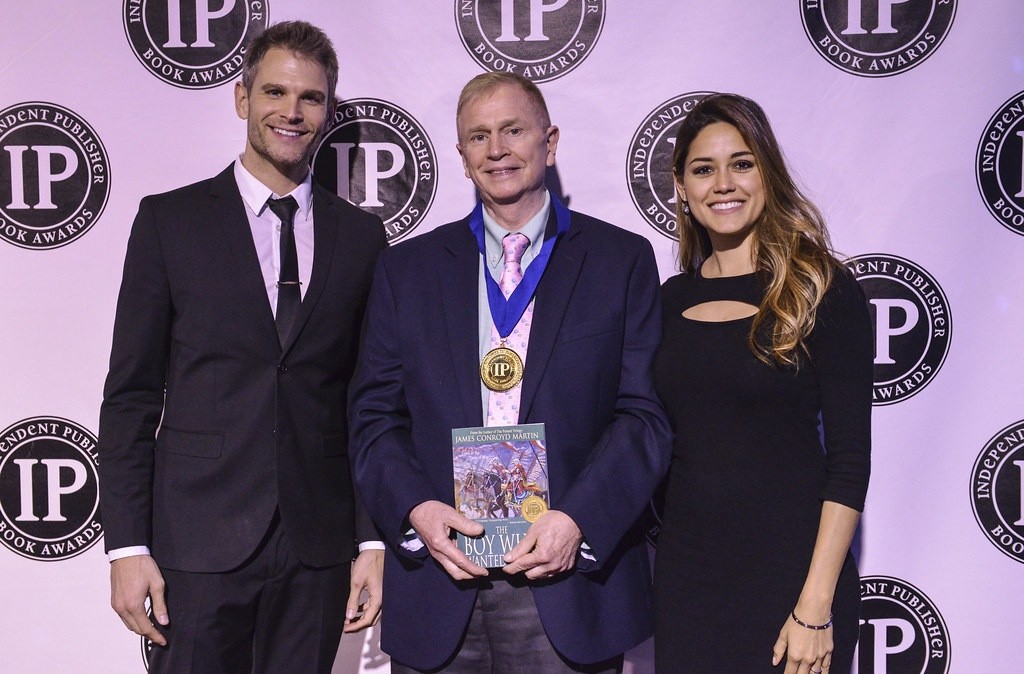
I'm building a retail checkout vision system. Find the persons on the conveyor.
[99,22,874,674]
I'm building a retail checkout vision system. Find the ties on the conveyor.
[266,195,303,352]
[499,233,530,300]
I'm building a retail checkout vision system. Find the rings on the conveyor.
[811,667,822,673]
[548,574,553,578]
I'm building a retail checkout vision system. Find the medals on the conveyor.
[480,338,524,390]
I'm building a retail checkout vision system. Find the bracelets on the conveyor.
[791,608,834,630]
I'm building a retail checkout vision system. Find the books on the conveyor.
[452,423,550,568]
